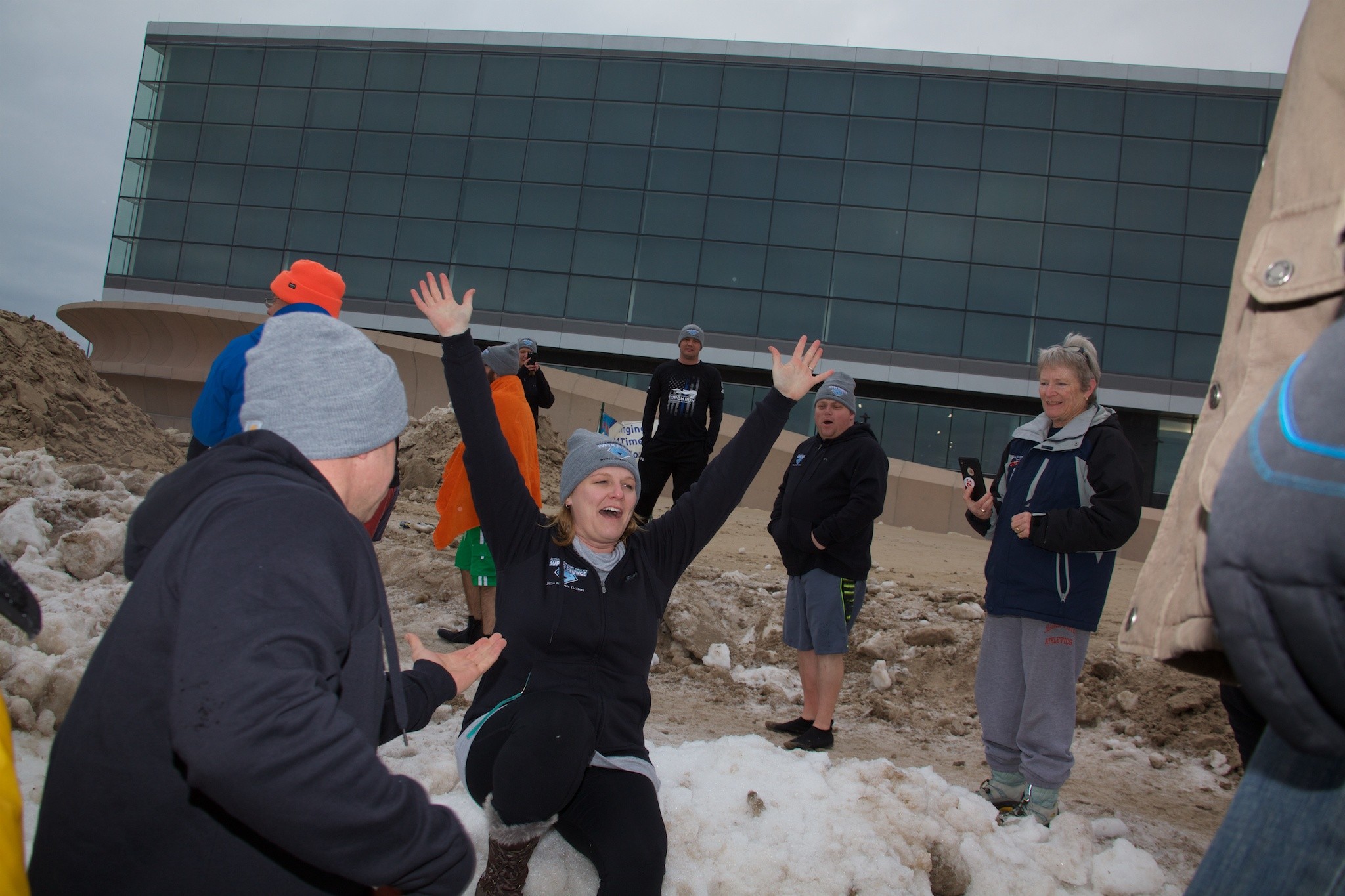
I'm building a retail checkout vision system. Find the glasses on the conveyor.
[1059,346,1088,360]
[265,297,281,307]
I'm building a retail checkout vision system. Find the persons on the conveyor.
[634,324,725,525]
[1186,315,1345,896]
[28,311,508,896]
[765,371,889,750]
[515,337,555,432]
[963,334,1145,828]
[411,271,835,896]
[186,260,345,461]
[433,342,543,645]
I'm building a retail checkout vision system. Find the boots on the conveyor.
[437,616,482,644]
[475,792,558,896]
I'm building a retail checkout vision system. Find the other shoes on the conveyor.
[765,716,813,736]
[783,720,834,749]
[995,795,1059,837]
[970,779,1027,809]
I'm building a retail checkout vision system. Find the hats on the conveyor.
[518,338,537,353]
[813,370,857,414]
[481,342,519,375]
[678,324,704,350]
[238,311,408,459]
[559,428,641,506]
[270,259,346,319]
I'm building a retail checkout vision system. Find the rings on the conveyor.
[1019,533,1022,537]
[980,508,985,512]
[1016,527,1019,532]
[808,366,814,371]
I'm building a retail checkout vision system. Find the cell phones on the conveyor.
[959,457,986,502]
[528,352,537,366]
